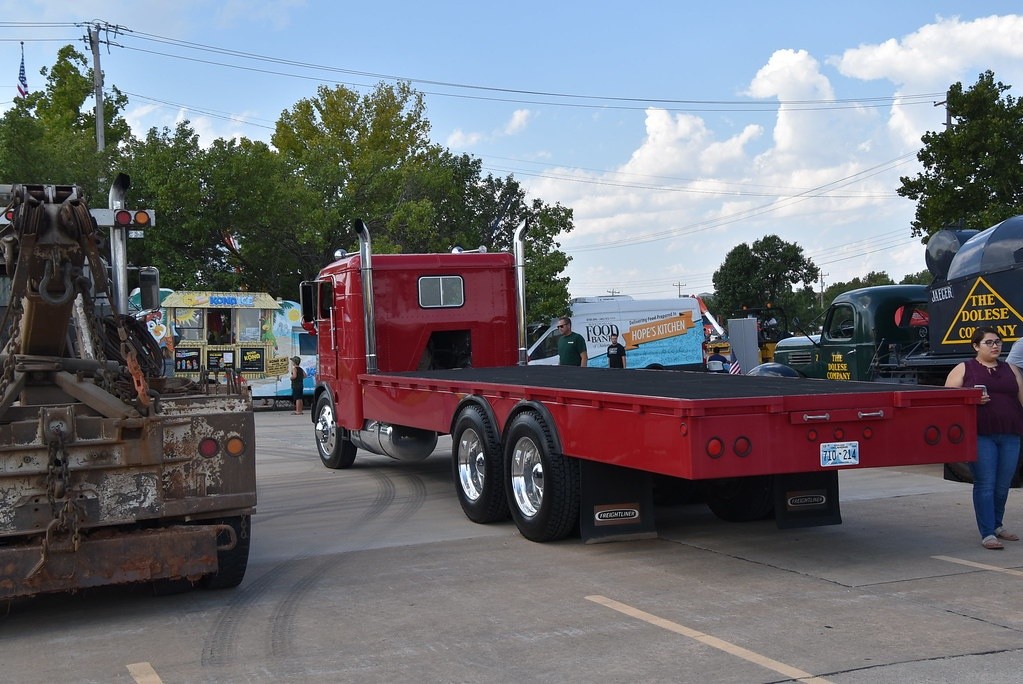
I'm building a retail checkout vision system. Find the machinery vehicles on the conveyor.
[0,171,260,600]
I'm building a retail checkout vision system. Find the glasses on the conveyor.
[979,339,1003,346]
[557,322,568,328]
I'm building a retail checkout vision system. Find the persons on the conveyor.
[557,317,587,367]
[1004,337,1023,406]
[708,347,730,373]
[945,327,1023,549]
[607,334,626,369]
[290,356,307,415]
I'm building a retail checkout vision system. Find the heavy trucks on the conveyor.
[298,218,984,545]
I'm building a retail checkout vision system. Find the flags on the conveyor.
[729,351,741,374]
[17,53,28,99]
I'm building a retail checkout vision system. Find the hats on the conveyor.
[290,356,301,365]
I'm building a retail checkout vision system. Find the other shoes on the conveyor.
[292,412,303,415]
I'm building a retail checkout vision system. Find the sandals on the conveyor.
[996,526,1019,540]
[981,534,1004,549]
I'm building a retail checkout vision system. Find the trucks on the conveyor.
[773,213,1023,488]
[527,297,707,371]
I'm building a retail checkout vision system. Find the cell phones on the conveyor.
[974,384,988,400]
